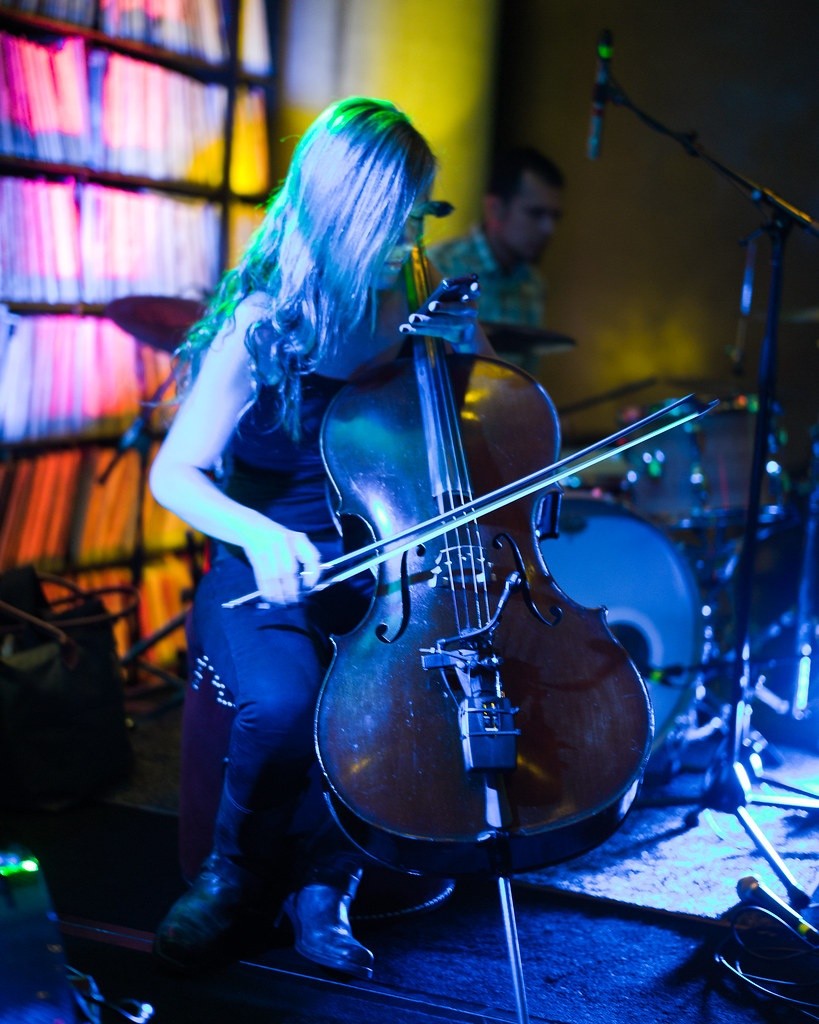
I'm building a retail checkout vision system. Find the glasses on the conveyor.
[510,199,564,222]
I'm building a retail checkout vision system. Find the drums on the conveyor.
[530,489,711,793]
[613,398,788,530]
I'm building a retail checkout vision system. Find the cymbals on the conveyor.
[478,319,586,349]
[107,295,204,355]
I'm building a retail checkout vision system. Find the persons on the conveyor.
[148,94,499,981]
[424,145,566,375]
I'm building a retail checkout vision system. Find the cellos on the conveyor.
[315,242,659,1024]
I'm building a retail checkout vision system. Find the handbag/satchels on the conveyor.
[0,565,141,807]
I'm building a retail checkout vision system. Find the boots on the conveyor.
[280,834,375,981]
[155,768,270,949]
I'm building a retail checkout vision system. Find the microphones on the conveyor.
[736,875,819,945]
[586,29,613,161]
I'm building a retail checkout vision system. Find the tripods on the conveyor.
[613,84,819,907]
